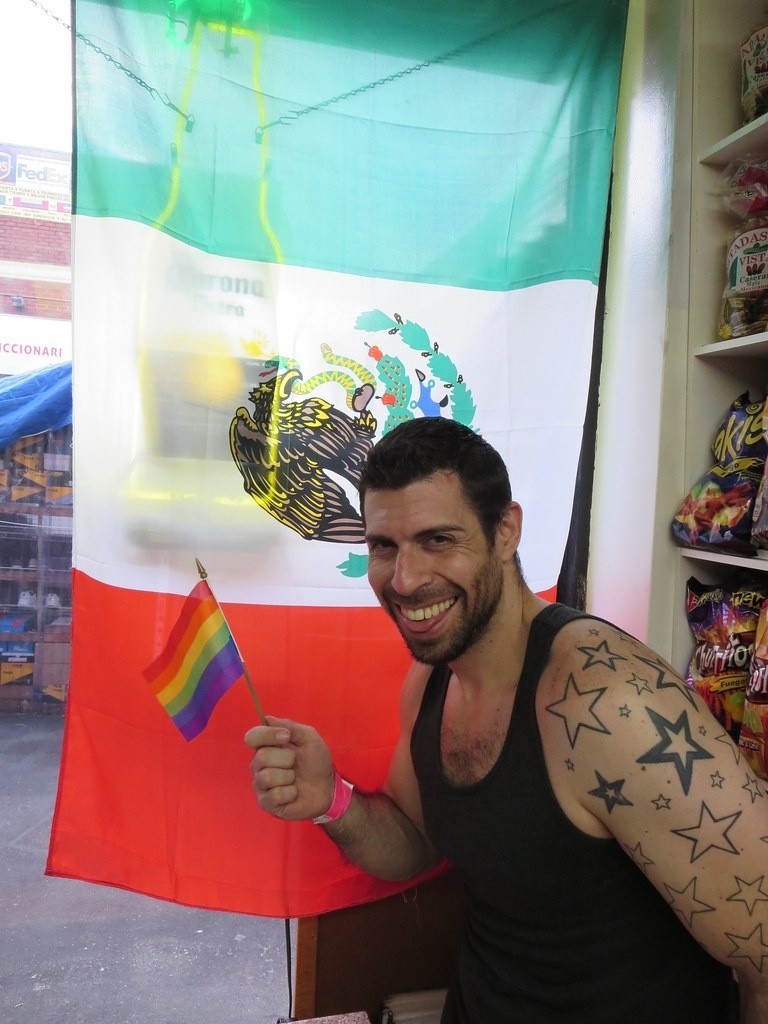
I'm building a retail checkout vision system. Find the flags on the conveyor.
[46,0,628,918]
[140,579,246,744]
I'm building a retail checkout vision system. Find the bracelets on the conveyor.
[313,767,354,824]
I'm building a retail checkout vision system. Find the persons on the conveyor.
[244,416,768,1024]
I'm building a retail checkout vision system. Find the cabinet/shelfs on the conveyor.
[0,503,73,715]
[676,0,768,787]
